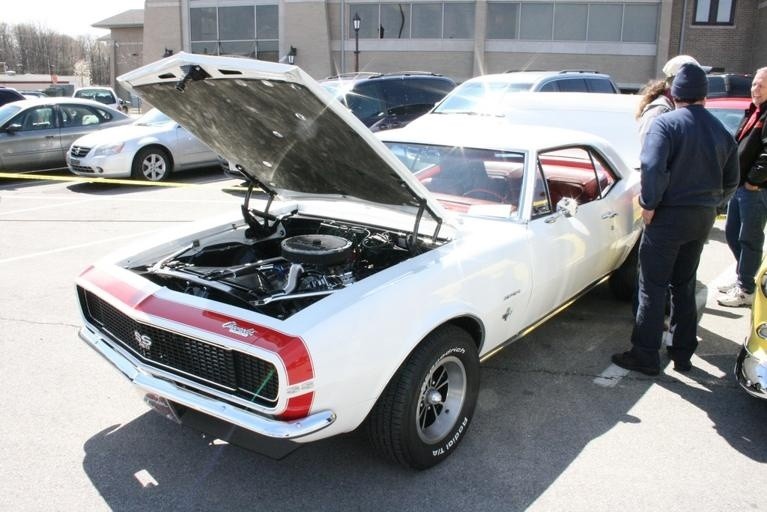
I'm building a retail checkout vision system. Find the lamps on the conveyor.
[287,45,298,63]
[160,48,173,58]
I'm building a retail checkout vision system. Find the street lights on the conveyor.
[350,9,362,72]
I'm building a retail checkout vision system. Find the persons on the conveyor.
[608,63,742,378]
[635,51,715,334]
[713,66,766,309]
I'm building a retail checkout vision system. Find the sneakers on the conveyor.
[612,352,659,374]
[718,282,754,307]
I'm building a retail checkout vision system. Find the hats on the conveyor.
[663,55,712,98]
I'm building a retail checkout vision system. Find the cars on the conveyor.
[728,250,766,402]
[67,46,663,478]
